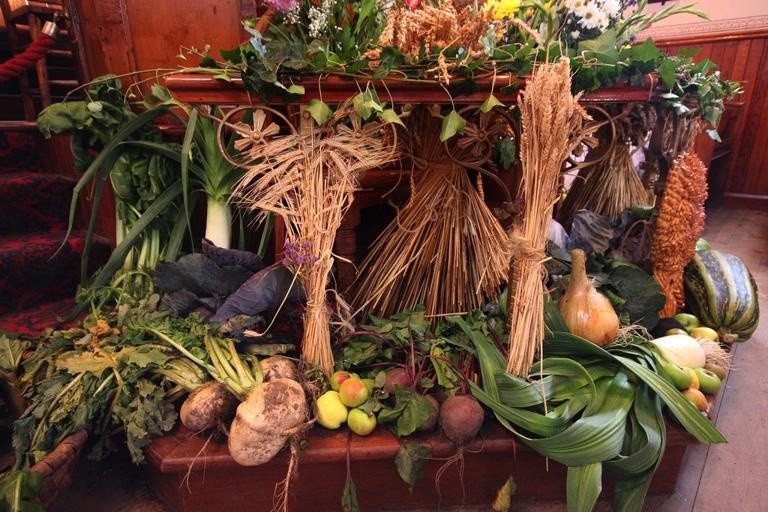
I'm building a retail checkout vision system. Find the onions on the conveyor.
[556,250,620,346]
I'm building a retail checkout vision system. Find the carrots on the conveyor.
[1,427,94,511]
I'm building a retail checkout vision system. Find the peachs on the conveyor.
[315,369,377,434]
